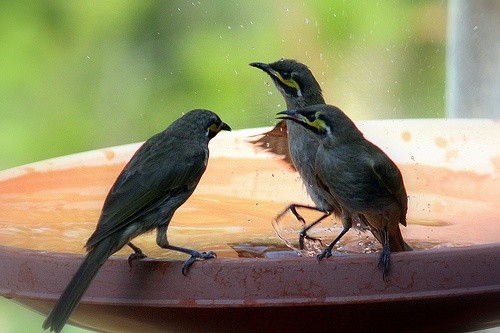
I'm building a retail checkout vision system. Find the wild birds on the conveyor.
[274,104,408,282]
[248,58,372,250]
[42,109,232,333]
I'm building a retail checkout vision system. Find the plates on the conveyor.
[0,119,500,333]
